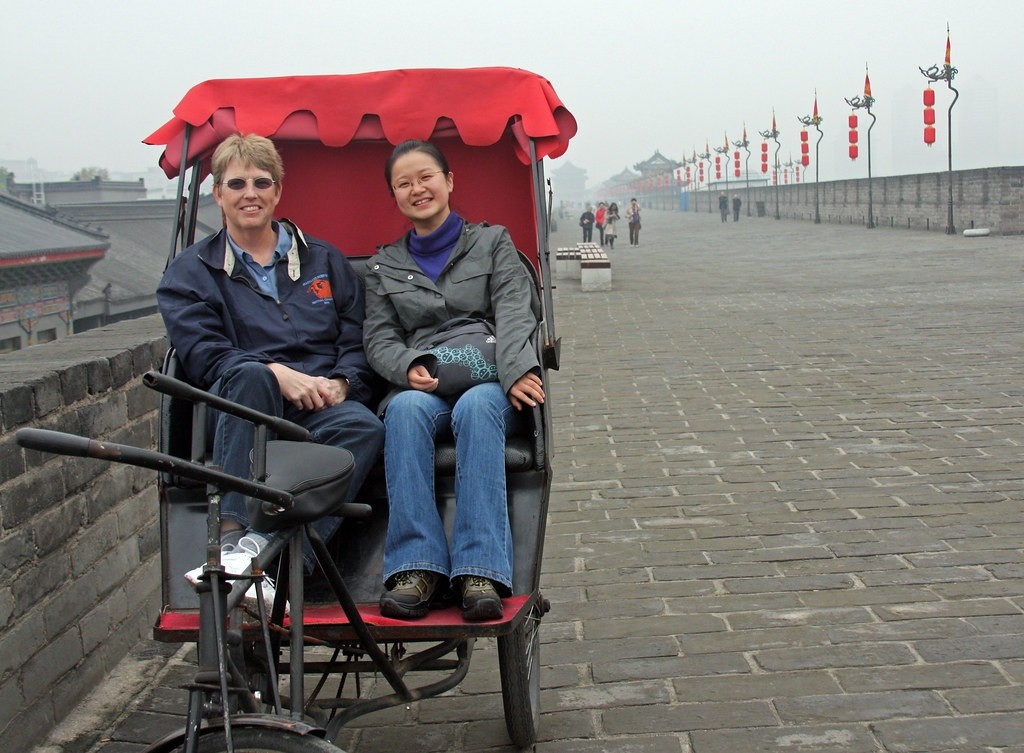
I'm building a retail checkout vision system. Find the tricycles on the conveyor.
[14,70,577,752]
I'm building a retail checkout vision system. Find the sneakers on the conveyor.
[460,574,504,622]
[183,537,290,626]
[378,569,451,620]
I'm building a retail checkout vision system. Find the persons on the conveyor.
[157,133,384,617]
[625,199,641,247]
[579,201,620,250]
[359,140,544,622]
[733,194,742,222]
[719,192,729,223]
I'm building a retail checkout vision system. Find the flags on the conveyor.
[682,136,729,162]
[813,100,818,116]
[864,75,871,96]
[743,128,747,138]
[773,116,776,128]
[945,38,950,63]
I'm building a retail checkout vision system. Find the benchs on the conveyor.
[158,248,548,611]
[556,242,612,292]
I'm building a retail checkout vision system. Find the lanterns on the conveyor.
[716,156,721,179]
[687,167,691,183]
[923,89,935,145]
[665,176,669,188]
[796,167,800,182]
[849,115,858,160]
[761,144,768,173]
[801,131,808,166]
[677,170,681,185]
[700,162,704,181]
[784,169,788,184]
[773,171,777,186]
[734,152,740,176]
[639,176,661,192]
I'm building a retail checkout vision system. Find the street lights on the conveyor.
[797,88,824,224]
[609,163,682,212]
[782,154,794,183]
[844,62,876,229]
[682,149,698,212]
[794,158,808,182]
[758,107,781,220]
[713,130,731,214]
[696,138,713,214]
[731,121,753,217]
[770,156,781,184]
[918,23,959,235]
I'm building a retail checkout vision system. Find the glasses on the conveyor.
[392,170,449,192]
[219,177,277,190]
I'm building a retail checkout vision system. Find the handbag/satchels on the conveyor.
[411,318,501,397]
[596,221,602,229]
[579,222,585,227]
